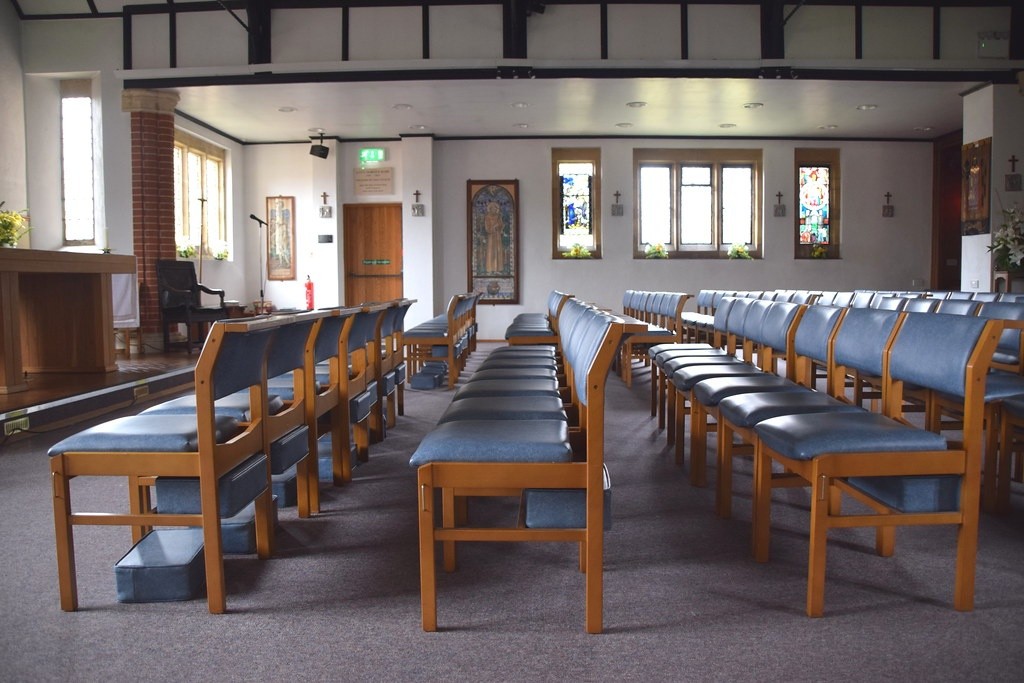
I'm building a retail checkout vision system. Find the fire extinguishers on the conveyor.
[305,275,314,310]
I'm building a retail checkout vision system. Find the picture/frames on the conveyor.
[466,178,520,305]
[960,136,992,223]
[265,195,296,280]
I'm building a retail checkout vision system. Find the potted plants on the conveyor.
[0,200,34,248]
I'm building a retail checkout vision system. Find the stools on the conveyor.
[112,327,143,358]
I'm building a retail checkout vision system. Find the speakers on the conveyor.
[310,145,330,158]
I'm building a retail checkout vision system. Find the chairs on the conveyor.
[46,287,1024,634]
[154,258,230,355]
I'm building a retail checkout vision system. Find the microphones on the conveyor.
[249,214,269,226]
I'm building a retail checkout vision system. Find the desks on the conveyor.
[0,248,138,396]
[993,270,1024,293]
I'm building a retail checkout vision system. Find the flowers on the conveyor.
[810,241,828,258]
[176,238,198,259]
[213,245,229,261]
[726,240,754,260]
[561,242,594,260]
[644,239,669,260]
[987,207,1024,274]
